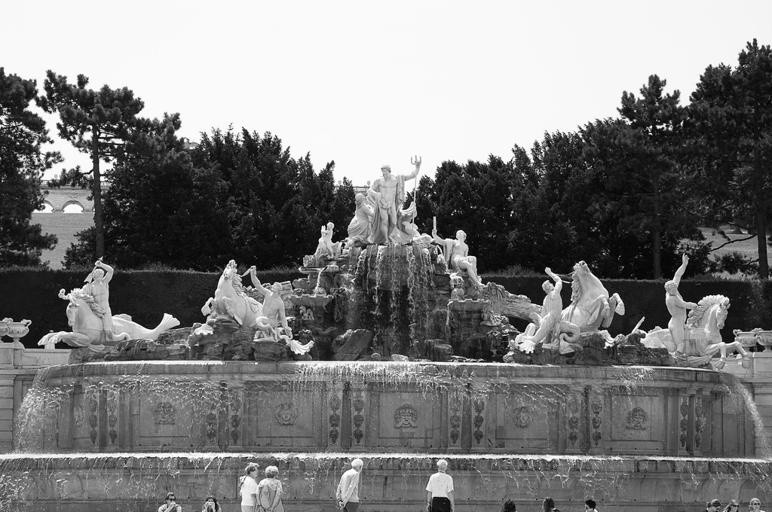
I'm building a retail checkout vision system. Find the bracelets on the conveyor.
[427,499,431,504]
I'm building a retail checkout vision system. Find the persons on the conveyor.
[318,222,334,260]
[239,461,260,511]
[257,465,285,511]
[723,498,741,512]
[522,266,563,343]
[704,498,721,511]
[158,491,181,511]
[432,229,485,288]
[426,458,456,511]
[748,497,766,511]
[541,496,562,512]
[251,265,293,342]
[202,495,221,510]
[665,253,697,356]
[500,500,518,512]
[348,161,421,246]
[84,259,129,341]
[584,499,599,511]
[335,458,364,510]
